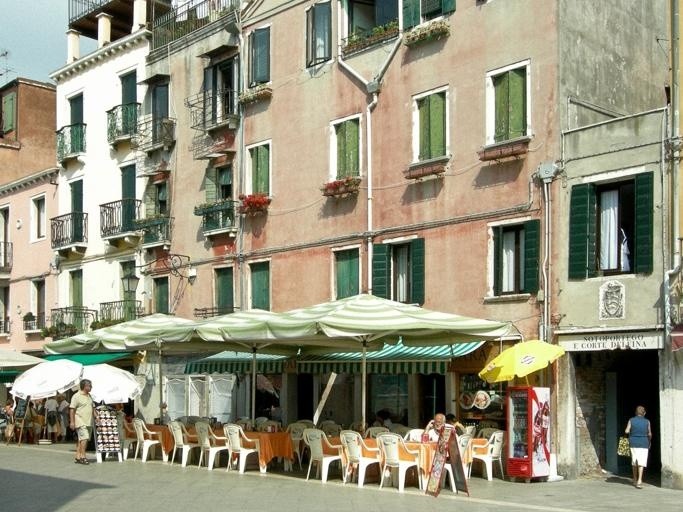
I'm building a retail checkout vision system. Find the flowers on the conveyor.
[324,175,353,191]
[236,192,268,215]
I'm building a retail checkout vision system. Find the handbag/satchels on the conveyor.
[617,437,630,457]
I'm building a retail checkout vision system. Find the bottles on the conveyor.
[514,393,528,458]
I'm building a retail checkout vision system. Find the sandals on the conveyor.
[74,458,83,463]
[636,483,642,488]
[81,458,89,465]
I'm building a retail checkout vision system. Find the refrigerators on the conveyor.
[504,385,551,477]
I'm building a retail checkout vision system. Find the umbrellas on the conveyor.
[479,339,565,387]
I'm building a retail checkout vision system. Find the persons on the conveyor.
[69,379,94,465]
[625,405,652,488]
[421,407,467,491]
